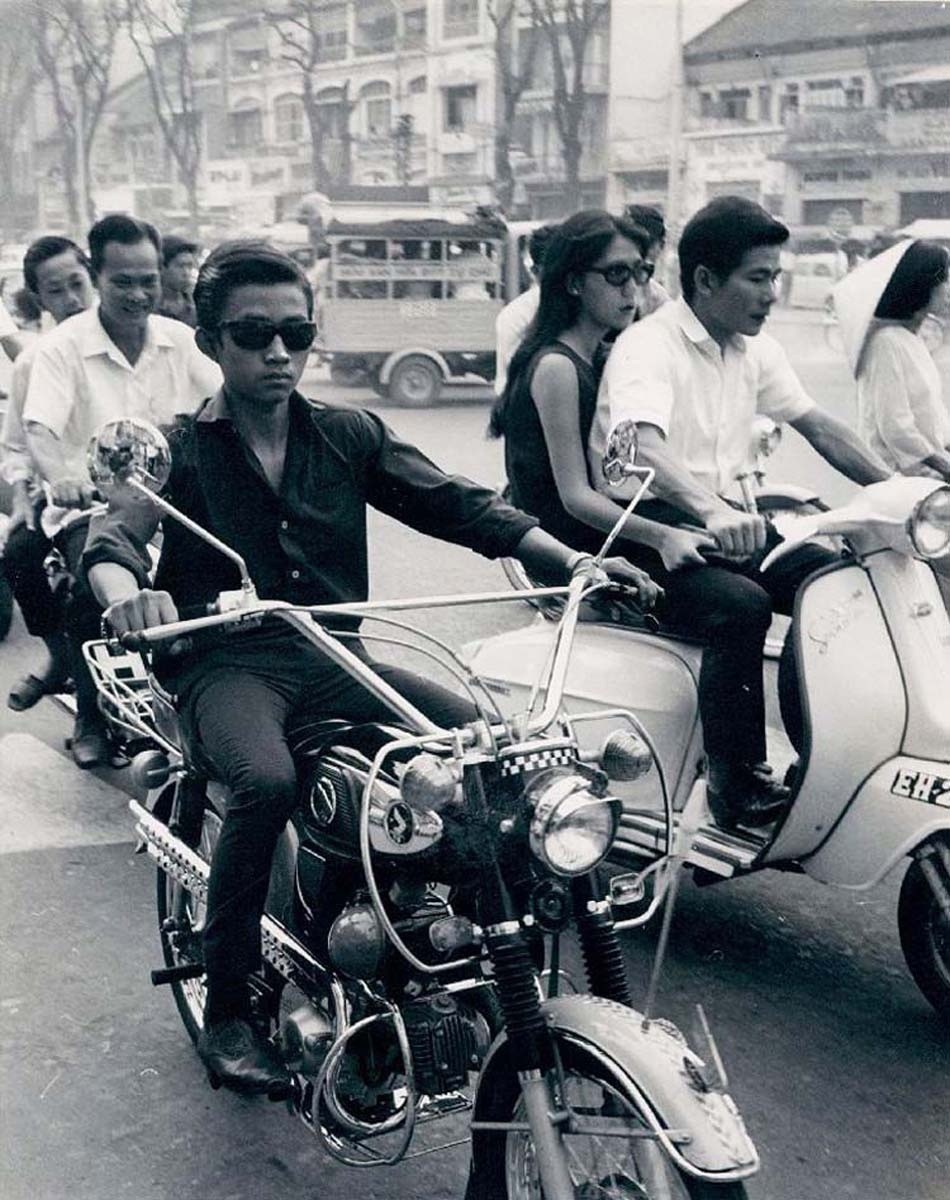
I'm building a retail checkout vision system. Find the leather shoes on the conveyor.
[707,771,794,829]
[783,764,801,788]
[197,1017,289,1094]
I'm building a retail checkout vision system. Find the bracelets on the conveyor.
[564,551,593,583]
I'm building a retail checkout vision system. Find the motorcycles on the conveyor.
[80,417,767,1200]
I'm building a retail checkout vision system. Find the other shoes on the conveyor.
[71,714,106,767]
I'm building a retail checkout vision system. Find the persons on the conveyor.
[484,194,895,829]
[0,213,225,769]
[81,241,666,1097]
[312,234,534,300]
[827,237,950,619]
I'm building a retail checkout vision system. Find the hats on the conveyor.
[831,236,917,379]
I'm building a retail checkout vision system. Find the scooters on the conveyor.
[433,453,950,1020]
[24,492,168,767]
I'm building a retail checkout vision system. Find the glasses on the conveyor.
[209,318,317,351]
[582,262,655,287]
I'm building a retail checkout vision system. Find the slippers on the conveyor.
[7,674,70,711]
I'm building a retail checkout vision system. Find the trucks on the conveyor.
[295,189,676,412]
